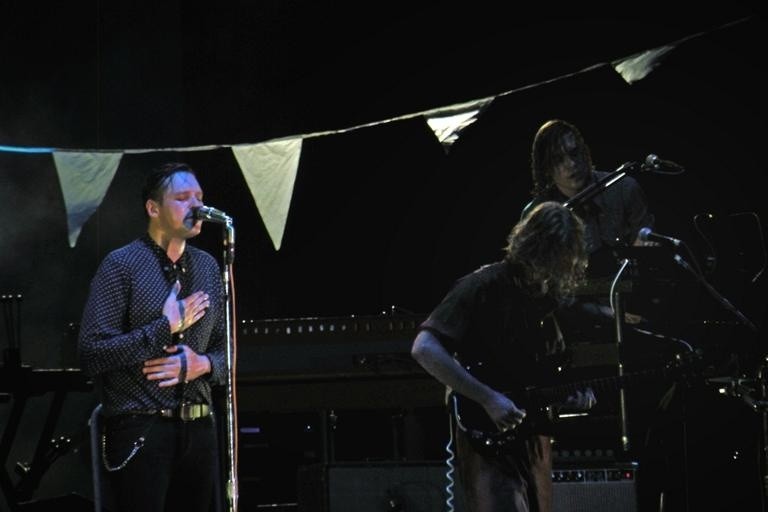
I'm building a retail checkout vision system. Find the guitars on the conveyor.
[447,344,705,448]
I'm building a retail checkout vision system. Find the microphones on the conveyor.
[639,228,682,248]
[193,205,232,225]
[645,154,683,170]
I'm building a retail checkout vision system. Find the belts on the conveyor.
[125,400,211,423]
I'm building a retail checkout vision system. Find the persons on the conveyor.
[77,163,234,512]
[411,202,587,512]
[520,116,654,462]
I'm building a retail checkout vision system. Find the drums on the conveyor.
[635,390,768,510]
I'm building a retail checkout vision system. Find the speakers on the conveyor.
[549,467,637,512]
[296,461,449,511]
